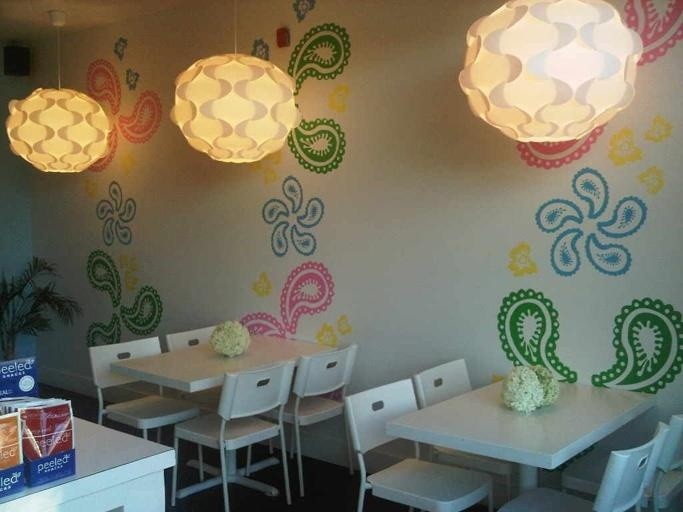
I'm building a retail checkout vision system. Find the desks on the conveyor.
[0,416,176,512]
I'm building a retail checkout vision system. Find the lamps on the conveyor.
[168,1,302,164]
[458,0,643,144]
[4,9,110,172]
